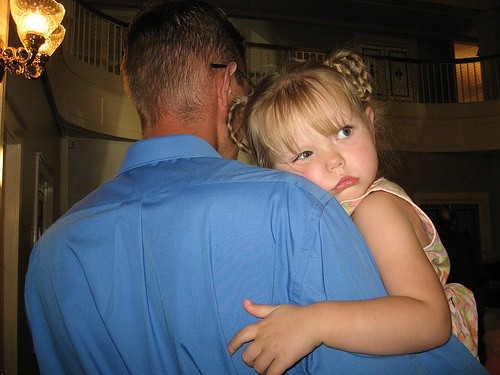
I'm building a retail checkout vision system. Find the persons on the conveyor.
[24,0,493,374]
[224,51,479,374]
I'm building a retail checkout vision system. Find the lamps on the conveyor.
[0,0,69,83]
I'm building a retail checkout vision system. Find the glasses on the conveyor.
[210,63,258,97]
